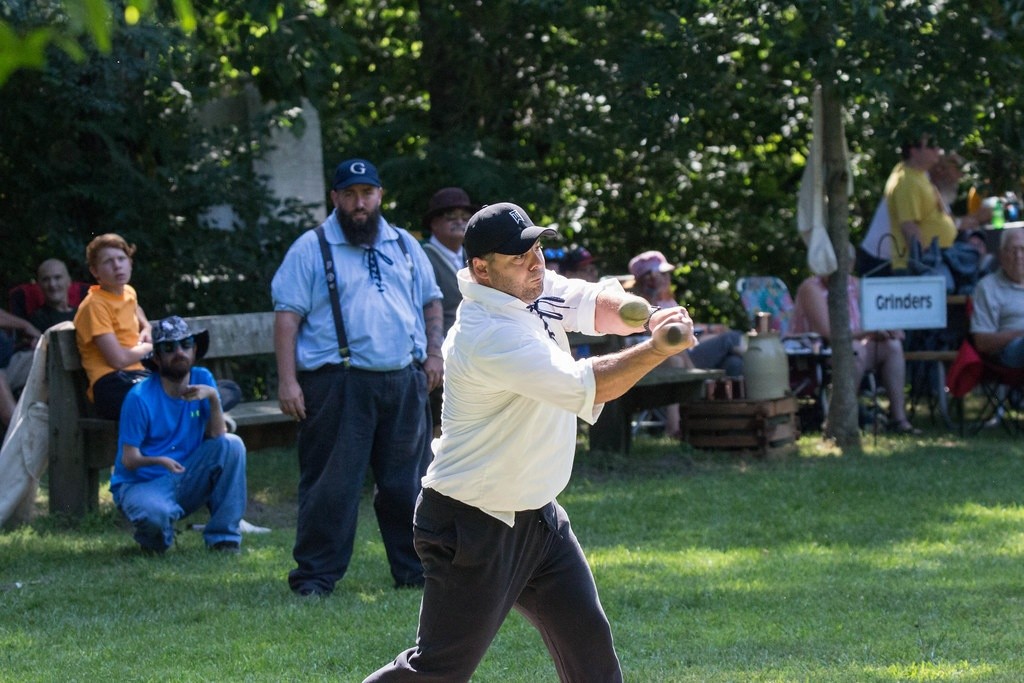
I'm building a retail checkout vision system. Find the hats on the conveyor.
[140,315,210,373]
[333,159,383,190]
[954,229,990,243]
[629,250,675,275]
[558,246,600,268]
[463,203,557,257]
[423,187,471,223]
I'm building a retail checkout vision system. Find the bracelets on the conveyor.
[643,305,662,334]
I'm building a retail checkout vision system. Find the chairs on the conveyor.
[735,289,1024,437]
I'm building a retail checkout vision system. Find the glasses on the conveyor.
[925,136,939,149]
[161,339,193,353]
[442,211,471,221]
[544,248,564,259]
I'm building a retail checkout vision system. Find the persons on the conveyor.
[786,116,1024,437]
[0,260,78,427]
[544,249,749,441]
[271,161,444,596]
[360,203,696,682]
[73,233,270,534]
[108,315,247,554]
[418,189,473,338]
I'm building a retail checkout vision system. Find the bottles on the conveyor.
[743,312,795,443]
[233,361,269,400]
[700,375,745,401]
[966,181,1020,228]
[654,322,691,353]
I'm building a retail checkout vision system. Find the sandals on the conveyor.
[890,418,923,436]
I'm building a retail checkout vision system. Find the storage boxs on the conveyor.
[679,395,798,460]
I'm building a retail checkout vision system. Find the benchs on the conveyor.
[41,308,301,515]
[568,330,740,456]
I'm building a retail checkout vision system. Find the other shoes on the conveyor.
[662,430,682,440]
[237,519,272,535]
[223,413,237,433]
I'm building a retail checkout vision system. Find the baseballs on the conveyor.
[618,297,650,327]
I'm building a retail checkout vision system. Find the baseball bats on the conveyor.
[664,327,704,348]
